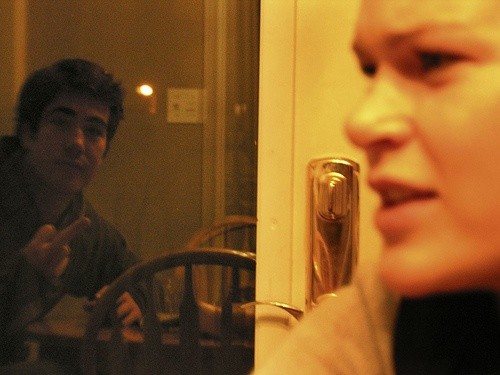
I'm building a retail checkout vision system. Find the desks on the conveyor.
[23,294,255,375]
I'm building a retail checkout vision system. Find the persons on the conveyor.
[0,56,168,375]
[247,0,500,375]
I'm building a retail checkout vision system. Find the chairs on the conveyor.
[79,248,256,375]
[167,216,257,333]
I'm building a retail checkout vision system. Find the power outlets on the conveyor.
[166,89,203,125]
